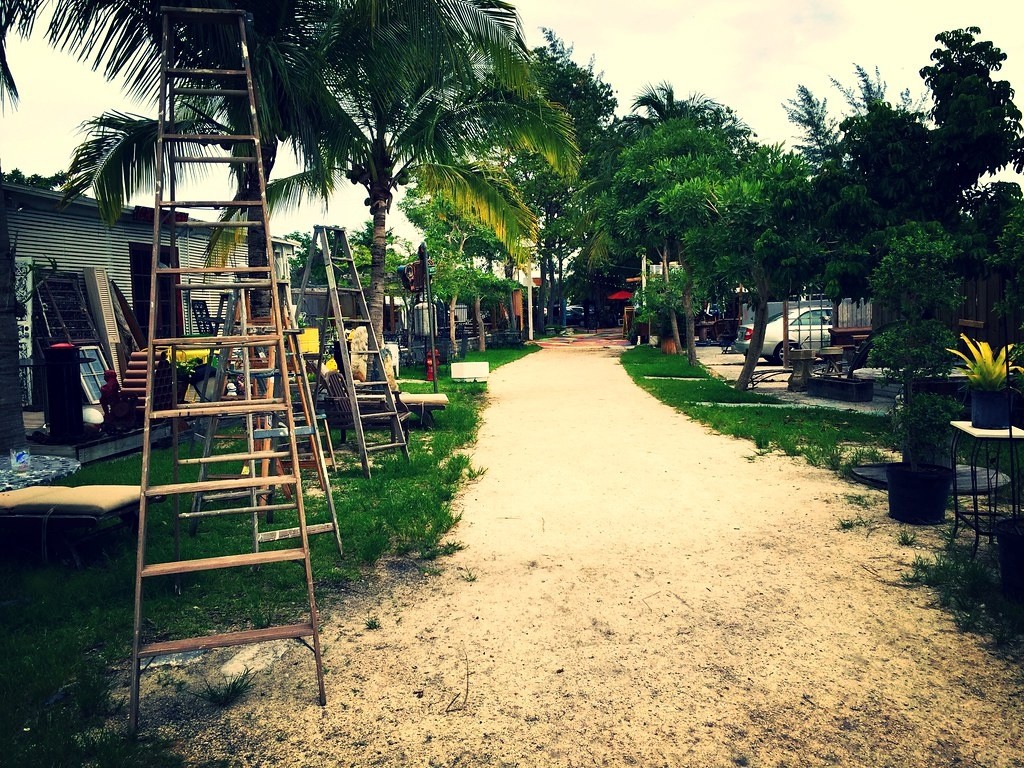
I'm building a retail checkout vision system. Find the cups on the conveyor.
[10,447,30,471]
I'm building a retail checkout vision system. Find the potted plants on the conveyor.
[948,334,1022,430]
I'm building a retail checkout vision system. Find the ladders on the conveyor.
[292,225,411,482]
[188,281,345,559]
[126,4,326,738]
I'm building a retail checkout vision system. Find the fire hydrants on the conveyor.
[426,348,440,381]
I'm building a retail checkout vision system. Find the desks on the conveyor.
[947,421,1024,563]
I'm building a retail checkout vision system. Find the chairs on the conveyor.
[321,372,412,451]
[301,360,431,395]
[0,477,165,562]
[336,389,450,428]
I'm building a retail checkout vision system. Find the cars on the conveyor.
[565,305,619,328]
[734,304,833,368]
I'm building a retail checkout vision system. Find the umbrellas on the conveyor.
[608,290,633,299]
